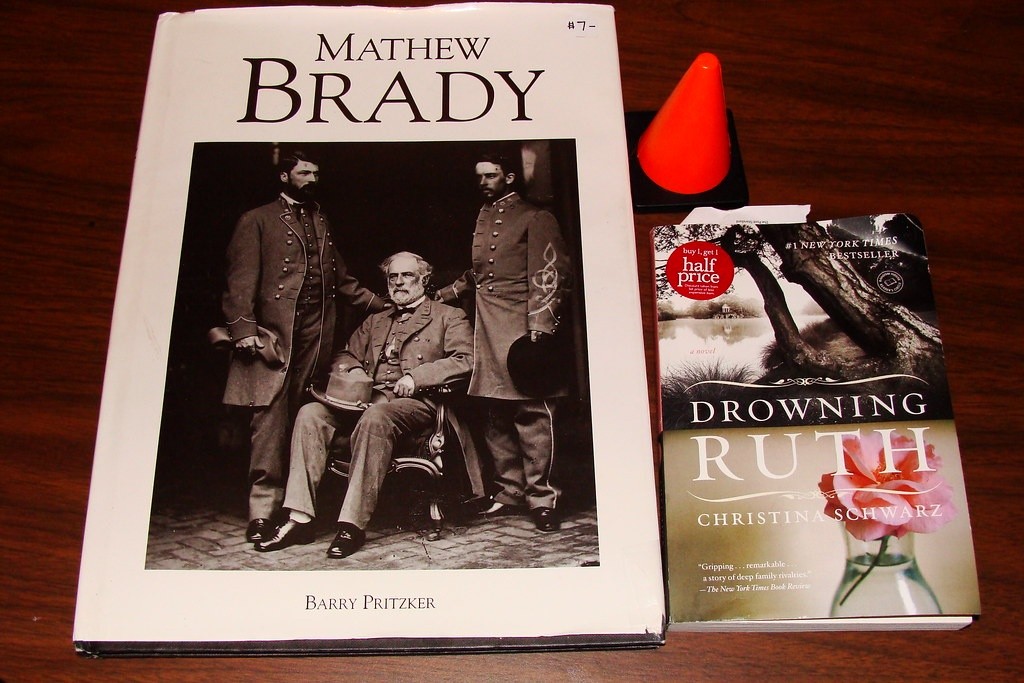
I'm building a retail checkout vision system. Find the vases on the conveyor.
[830,518,944,615]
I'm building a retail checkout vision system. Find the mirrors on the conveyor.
[327,376,473,540]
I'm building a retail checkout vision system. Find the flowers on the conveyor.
[821,429,957,540]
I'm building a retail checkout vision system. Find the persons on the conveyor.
[254,252,474,559]
[221,151,392,542]
[434,154,571,531]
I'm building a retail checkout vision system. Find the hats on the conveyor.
[310,370,388,412]
[208,322,285,369]
[507,332,573,399]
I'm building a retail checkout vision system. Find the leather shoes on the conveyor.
[254,520,317,552]
[532,506,560,531]
[246,518,273,543]
[477,500,527,516]
[326,522,365,558]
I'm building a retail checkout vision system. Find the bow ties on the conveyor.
[396,306,415,316]
[293,201,309,209]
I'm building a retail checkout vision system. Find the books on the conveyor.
[653,213,981,632]
[74,3,667,658]
